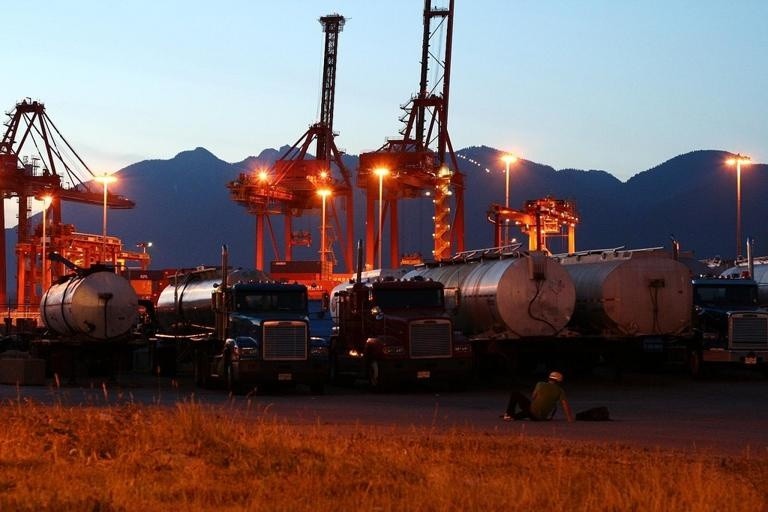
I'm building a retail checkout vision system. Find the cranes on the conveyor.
[354,1,466,271]
[0,100,137,312]
[226,15,353,300]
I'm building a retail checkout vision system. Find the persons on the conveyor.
[503,371,574,422]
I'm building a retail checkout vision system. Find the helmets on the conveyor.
[548,371,564,384]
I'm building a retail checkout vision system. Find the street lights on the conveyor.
[40,194,52,298]
[93,175,118,264]
[316,169,332,274]
[724,154,751,258]
[500,153,517,246]
[374,168,389,269]
